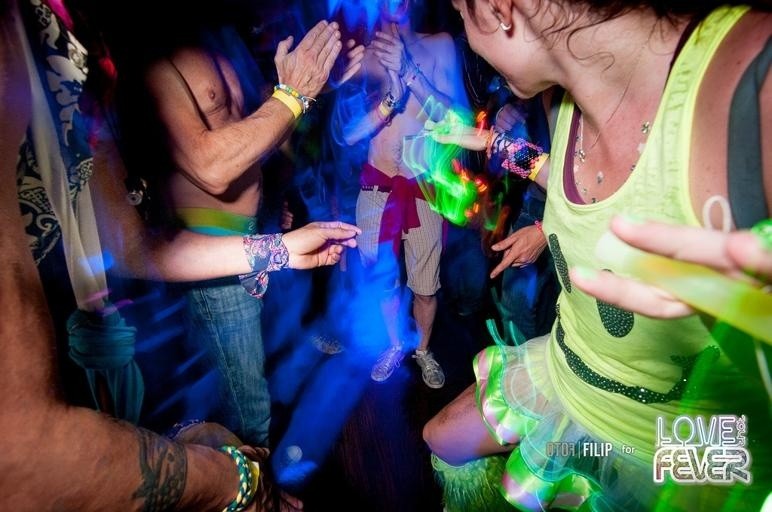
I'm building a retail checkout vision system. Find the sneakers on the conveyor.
[414,348,448,390]
[371,342,409,383]
[310,330,347,354]
